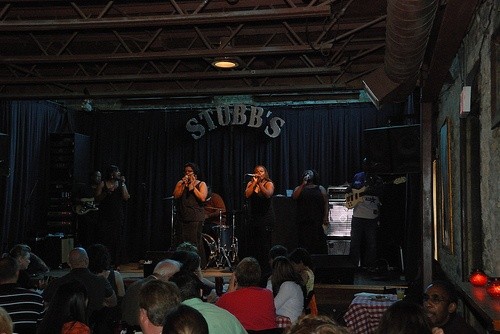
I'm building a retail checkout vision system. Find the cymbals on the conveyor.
[206,206,225,211]
[160,196,174,201]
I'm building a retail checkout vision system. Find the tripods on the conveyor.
[204,211,238,270]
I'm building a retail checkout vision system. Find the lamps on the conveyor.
[81,98,96,112]
[211,36,240,69]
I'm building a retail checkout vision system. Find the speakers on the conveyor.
[48,132,90,183]
[144,251,175,278]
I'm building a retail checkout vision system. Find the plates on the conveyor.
[369,296,390,301]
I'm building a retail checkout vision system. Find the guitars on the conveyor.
[345,176,407,211]
[75,194,130,215]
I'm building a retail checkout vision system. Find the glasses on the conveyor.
[423,295,449,304]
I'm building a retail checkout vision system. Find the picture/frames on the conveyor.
[491,22,500,130]
[439,116,455,256]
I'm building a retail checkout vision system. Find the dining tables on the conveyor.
[343,291,407,334]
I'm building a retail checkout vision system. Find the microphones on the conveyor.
[246,173,258,177]
[182,175,188,185]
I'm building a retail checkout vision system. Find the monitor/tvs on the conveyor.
[361,124,420,176]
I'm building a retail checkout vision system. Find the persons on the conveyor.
[172,162,208,270]
[266,245,289,293]
[0,243,226,334]
[288,248,314,308]
[95,164,129,271]
[215,257,278,334]
[244,165,275,251]
[292,168,329,254]
[346,152,387,278]
[201,184,226,262]
[70,169,101,244]
[271,255,307,328]
[161,300,432,334]
[420,279,479,334]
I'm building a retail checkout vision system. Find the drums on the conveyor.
[210,226,233,253]
[201,233,217,264]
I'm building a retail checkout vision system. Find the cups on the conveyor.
[397,288,404,299]
[287,190,293,197]
